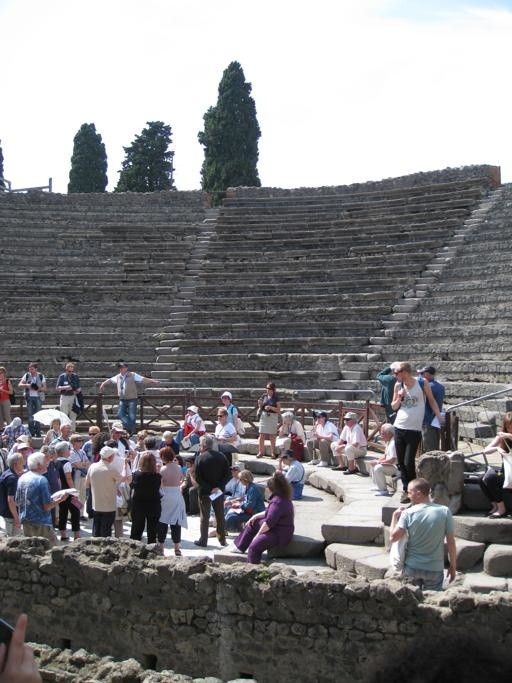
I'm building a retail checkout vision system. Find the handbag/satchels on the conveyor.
[9,389,16,405]
[501,451,512,489]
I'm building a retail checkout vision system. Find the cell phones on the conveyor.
[0,618,16,656]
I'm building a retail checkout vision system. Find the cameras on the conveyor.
[32,383,38,390]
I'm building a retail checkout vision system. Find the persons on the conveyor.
[0,614,42,683]
[223,471,294,564]
[265,450,305,503]
[100,363,160,438]
[220,391,239,434]
[0,367,14,426]
[307,412,339,467]
[56,362,83,434]
[367,423,399,497]
[377,363,406,425]
[0,404,263,556]
[391,362,447,503]
[275,411,305,461]
[479,412,512,517]
[18,363,48,438]
[330,412,367,474]
[387,476,458,595]
[255,382,281,461]
[416,365,446,451]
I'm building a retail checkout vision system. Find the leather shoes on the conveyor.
[332,466,345,471]
[486,511,507,519]
[343,467,358,474]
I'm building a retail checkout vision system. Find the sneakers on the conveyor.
[316,461,328,467]
[374,490,389,496]
[195,541,207,546]
[307,459,320,464]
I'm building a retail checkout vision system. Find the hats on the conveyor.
[112,422,126,432]
[221,391,232,400]
[185,405,198,413]
[316,411,328,417]
[417,366,435,375]
[12,417,29,450]
[343,413,357,419]
[99,446,119,459]
